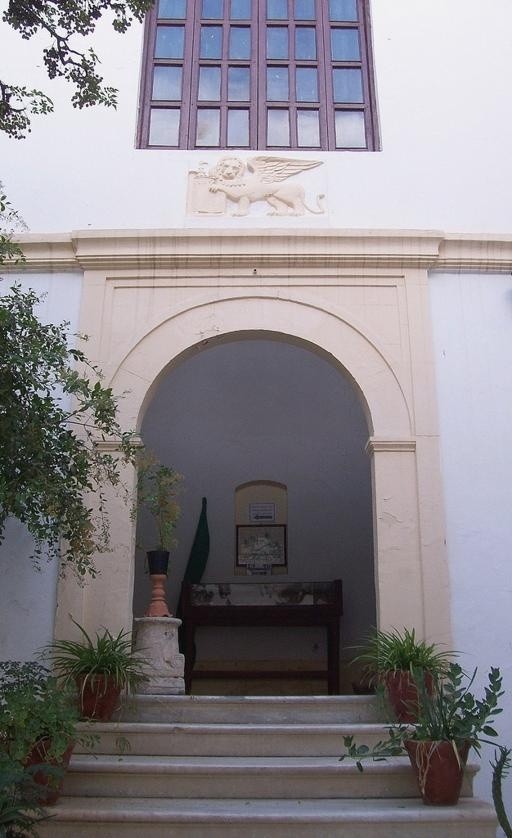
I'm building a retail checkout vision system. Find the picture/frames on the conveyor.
[234,521,288,569]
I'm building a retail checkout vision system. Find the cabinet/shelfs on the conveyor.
[174,573,344,701]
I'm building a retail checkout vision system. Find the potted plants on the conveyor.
[341,621,476,725]
[117,454,187,576]
[31,613,163,729]
[333,661,507,808]
[0,656,101,810]
